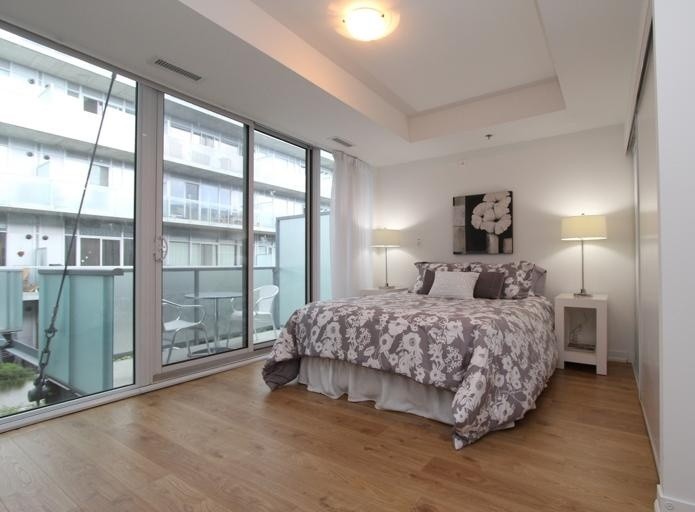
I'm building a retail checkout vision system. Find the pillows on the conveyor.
[411,260,547,301]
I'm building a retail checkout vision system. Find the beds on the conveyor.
[297,288,555,433]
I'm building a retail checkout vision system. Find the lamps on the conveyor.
[560,213,608,297]
[368,228,401,288]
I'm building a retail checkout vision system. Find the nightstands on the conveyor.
[555,293,609,376]
[360,288,408,297]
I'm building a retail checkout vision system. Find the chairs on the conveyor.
[161,284,279,365]
